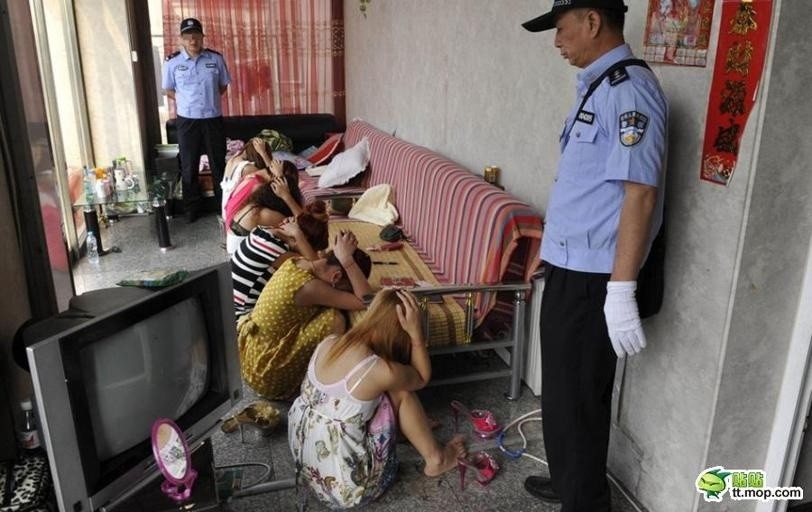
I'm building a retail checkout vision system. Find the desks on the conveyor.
[46,435,223,510]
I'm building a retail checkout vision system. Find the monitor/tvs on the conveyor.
[26,262,243,512]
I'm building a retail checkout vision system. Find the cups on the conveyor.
[94,178,111,198]
[114,157,135,194]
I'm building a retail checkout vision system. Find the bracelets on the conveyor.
[344,261,355,269]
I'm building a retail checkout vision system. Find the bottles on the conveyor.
[85,231,99,265]
[81,166,93,200]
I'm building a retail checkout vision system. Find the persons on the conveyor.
[219,137,273,220]
[223,158,299,234]
[229,201,329,336]
[173,336,208,423]
[287,288,466,509]
[162,19,231,224]
[521,0,671,512]
[225,176,302,257]
[235,229,371,404]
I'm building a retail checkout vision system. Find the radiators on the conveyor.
[484,268,545,397]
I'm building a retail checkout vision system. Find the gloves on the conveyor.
[603,280,647,360]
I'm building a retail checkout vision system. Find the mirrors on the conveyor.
[150,417,201,503]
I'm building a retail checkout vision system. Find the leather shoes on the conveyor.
[525,476,561,503]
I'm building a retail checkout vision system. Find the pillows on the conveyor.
[317,135,372,189]
[305,131,344,166]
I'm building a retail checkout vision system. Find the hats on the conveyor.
[522,0,628,32]
[181,19,202,34]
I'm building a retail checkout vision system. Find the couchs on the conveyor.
[290,117,548,400]
[155,110,340,221]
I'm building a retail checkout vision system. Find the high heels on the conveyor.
[451,400,502,438]
[221,401,281,444]
[457,450,499,490]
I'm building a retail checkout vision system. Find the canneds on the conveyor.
[484,166,499,183]
